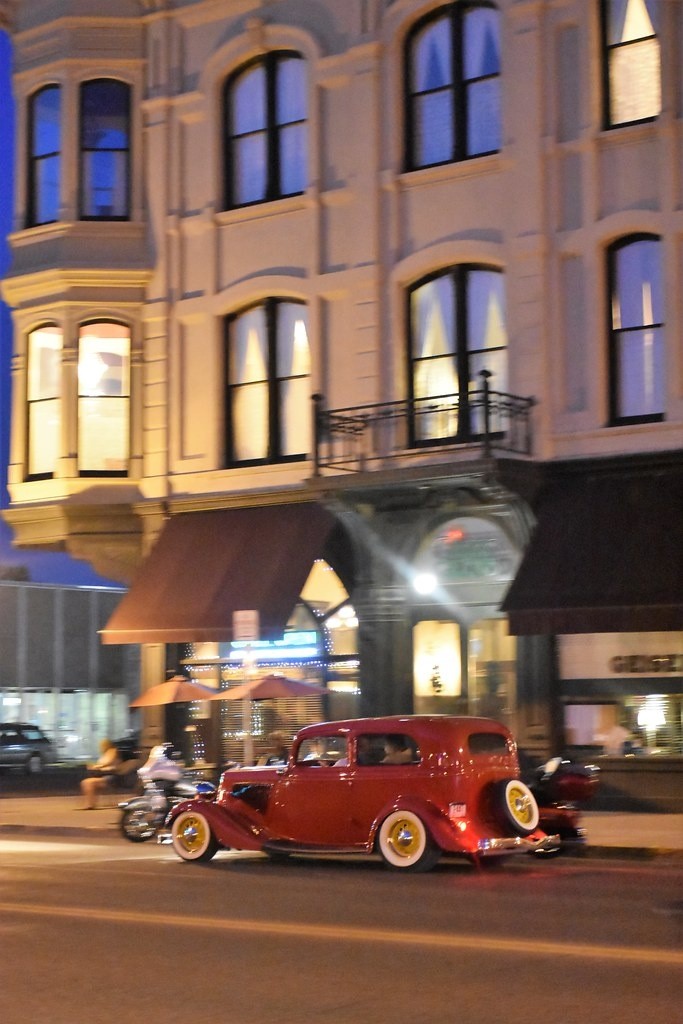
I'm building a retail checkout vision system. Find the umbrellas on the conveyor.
[128,674,218,756]
[212,674,333,732]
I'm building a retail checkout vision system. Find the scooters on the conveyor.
[116,767,219,844]
[520,756,599,858]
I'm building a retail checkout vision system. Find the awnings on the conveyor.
[94,502,336,643]
[496,464,682,638]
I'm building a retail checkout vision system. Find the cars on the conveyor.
[164,713,561,874]
[112,730,143,765]
[1,722,53,777]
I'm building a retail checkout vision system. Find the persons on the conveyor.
[77,738,121,810]
[255,732,412,768]
